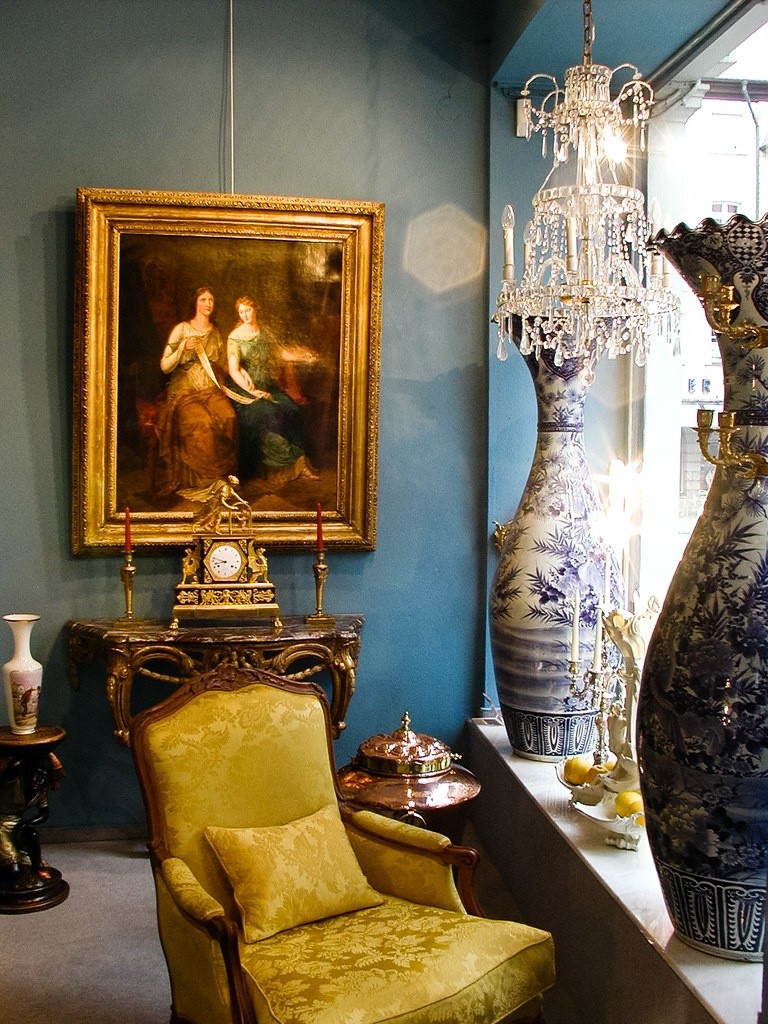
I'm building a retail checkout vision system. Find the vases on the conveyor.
[0,613,44,736]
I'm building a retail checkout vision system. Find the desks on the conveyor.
[64,610,367,754]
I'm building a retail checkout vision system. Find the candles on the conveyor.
[116,506,141,623]
[305,501,336,626]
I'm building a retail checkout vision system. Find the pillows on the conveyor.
[201,804,386,946]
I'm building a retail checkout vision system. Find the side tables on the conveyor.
[1,724,72,917]
[331,755,481,851]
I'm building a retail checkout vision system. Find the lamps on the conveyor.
[484,0,690,367]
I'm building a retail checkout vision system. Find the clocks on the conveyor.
[169,475,285,630]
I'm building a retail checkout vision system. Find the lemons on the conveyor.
[564,752,646,827]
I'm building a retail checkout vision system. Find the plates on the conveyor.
[554,757,605,810]
[568,792,647,851]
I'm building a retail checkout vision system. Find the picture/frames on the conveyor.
[67,186,387,557]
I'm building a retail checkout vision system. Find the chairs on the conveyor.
[126,651,558,1023]
